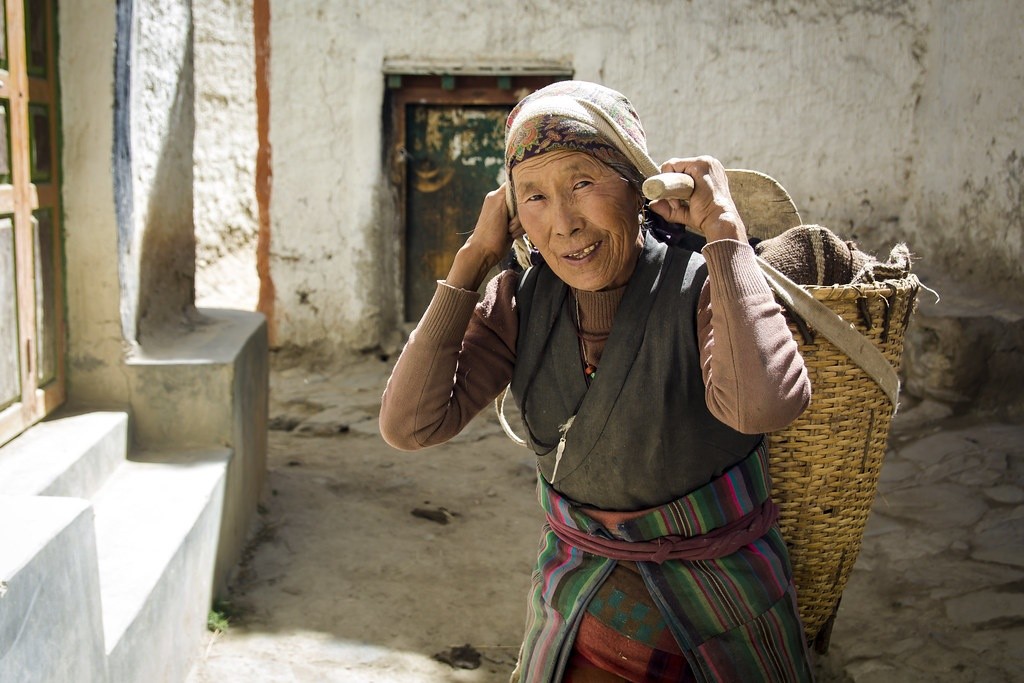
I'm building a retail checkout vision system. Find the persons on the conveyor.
[377,78,817,683]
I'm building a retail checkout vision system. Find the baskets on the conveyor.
[764,274,920,648]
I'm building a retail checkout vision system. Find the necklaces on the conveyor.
[574,286,597,379]
